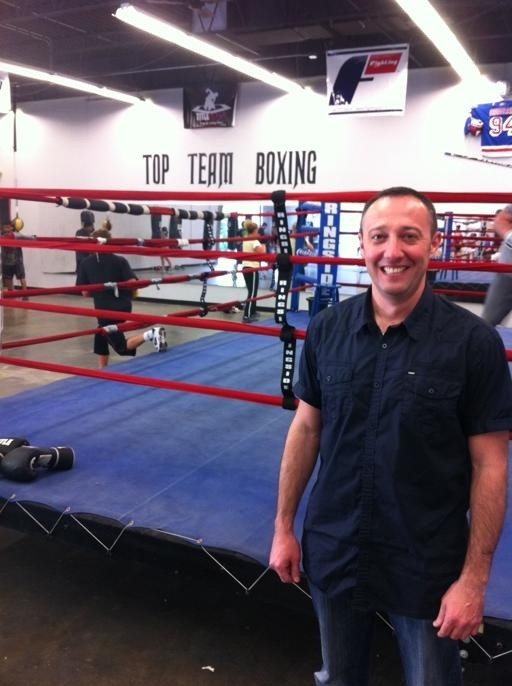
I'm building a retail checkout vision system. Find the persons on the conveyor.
[157,227,173,274]
[450,221,490,263]
[268,188,510,686]
[76,221,94,271]
[480,204,511,328]
[240,219,267,324]
[233,222,313,293]
[74,227,168,367]
[1,222,30,302]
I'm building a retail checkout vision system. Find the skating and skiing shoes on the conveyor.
[151,328,167,353]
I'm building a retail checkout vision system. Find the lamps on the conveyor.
[0,59,145,105]
[112,3,304,93]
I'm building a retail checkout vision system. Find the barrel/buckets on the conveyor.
[306,296,329,316]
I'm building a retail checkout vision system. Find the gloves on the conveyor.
[0,437,75,481]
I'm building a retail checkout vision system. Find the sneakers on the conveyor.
[243,312,260,323]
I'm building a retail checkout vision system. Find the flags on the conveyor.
[182,82,243,131]
[322,42,411,118]
